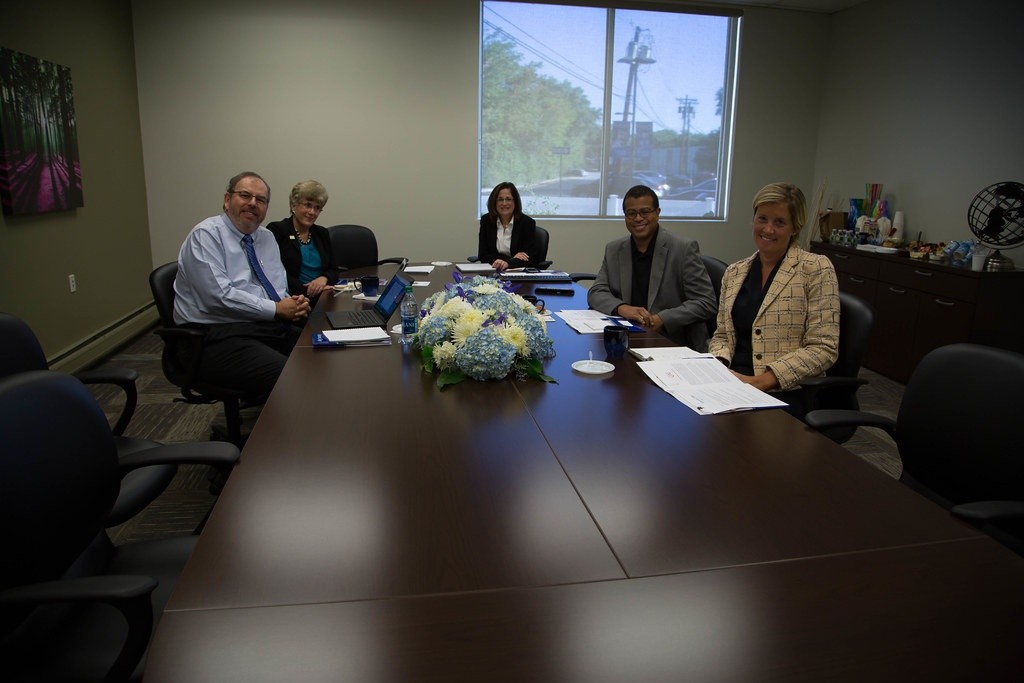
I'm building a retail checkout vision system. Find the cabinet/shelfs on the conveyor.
[810,241,1024,385]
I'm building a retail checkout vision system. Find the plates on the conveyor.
[431,260,452,266]
[333,284,360,290]
[876,246,898,253]
[571,359,615,375]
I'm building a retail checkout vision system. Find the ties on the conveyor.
[241,234,281,303]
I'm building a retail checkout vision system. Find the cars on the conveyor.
[569,168,717,200]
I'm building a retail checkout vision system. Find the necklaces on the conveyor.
[295,228,311,245]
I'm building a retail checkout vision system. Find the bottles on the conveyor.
[399,286,419,344]
[829,228,862,246]
[945,238,982,263]
[863,217,878,240]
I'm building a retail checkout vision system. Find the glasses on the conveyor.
[624,207,658,220]
[298,202,323,212]
[228,191,268,206]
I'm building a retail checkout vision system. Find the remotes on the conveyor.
[535,287,574,294]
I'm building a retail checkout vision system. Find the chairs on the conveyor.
[1,225,407,683]
[467,227,553,270]
[569,257,1024,560]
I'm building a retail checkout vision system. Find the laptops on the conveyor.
[325,269,414,329]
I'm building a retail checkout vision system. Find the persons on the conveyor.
[708,183,840,421]
[587,185,717,353]
[266,179,339,310]
[478,182,536,271]
[172,171,311,392]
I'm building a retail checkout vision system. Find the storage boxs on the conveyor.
[818,212,848,241]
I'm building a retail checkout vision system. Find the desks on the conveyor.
[145,262,1023,682]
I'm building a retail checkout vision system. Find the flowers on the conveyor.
[397,272,560,390]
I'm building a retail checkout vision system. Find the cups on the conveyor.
[971,255,985,271]
[603,326,630,356]
[353,276,380,296]
[891,210,904,242]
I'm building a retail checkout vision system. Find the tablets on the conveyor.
[399,258,407,271]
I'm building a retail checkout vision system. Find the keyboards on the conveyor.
[500,276,573,282]
